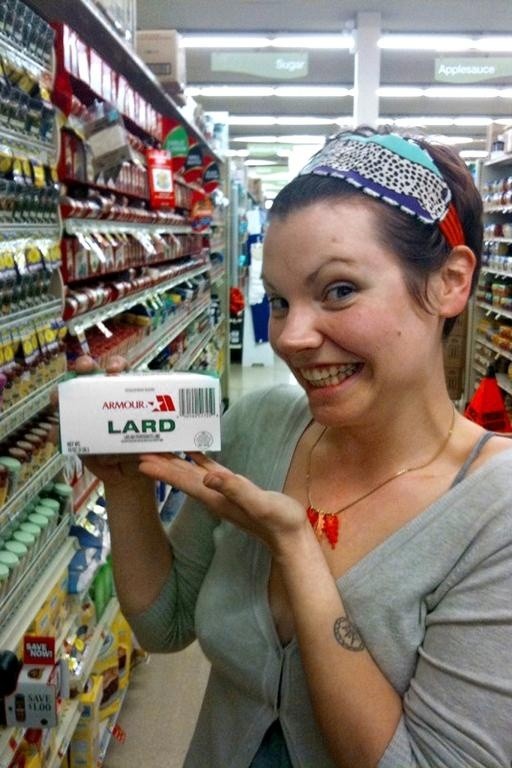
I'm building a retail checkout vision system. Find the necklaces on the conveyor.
[305,401,456,550]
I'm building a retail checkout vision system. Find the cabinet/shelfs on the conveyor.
[231,181,267,288]
[436,157,511,415]
[0,0,230,768]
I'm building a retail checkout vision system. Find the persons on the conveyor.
[49,123,512,768]
